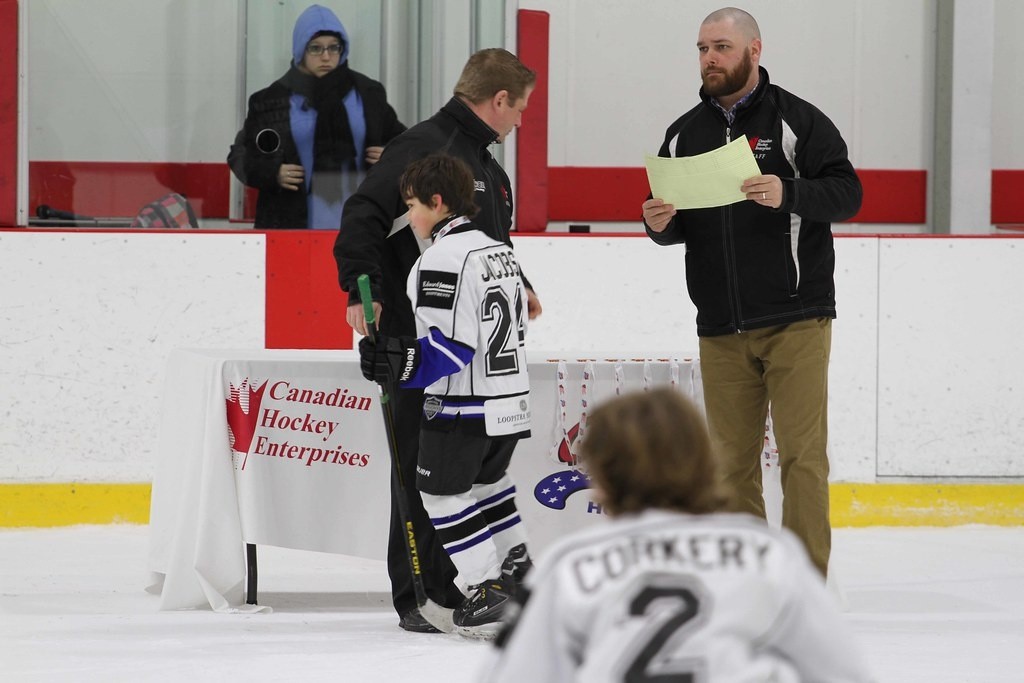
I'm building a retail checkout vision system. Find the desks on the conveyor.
[148,343,783,616]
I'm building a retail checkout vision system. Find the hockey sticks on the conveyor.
[358,274,458,635]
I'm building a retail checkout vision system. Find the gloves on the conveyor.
[359,336,421,383]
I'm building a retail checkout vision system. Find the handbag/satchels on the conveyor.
[131,191,198,228]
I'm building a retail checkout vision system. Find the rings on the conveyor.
[288,172,290,176]
[762,192,767,200]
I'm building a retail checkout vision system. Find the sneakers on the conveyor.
[453,580,513,641]
[502,544,533,600]
[398,608,441,632]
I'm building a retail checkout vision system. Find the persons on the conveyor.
[337,43,544,620]
[506,385,879,683]
[363,154,533,632]
[227,4,408,231]
[640,5,862,582]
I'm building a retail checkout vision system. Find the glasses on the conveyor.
[307,43,342,54]
